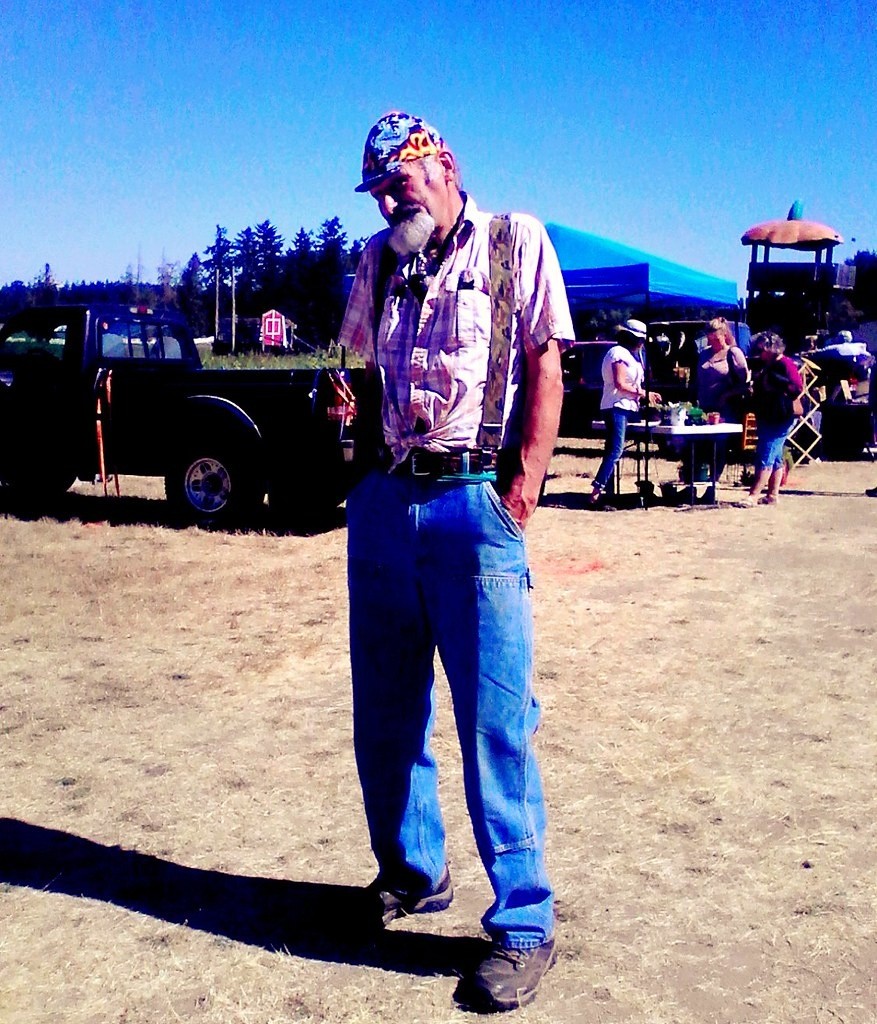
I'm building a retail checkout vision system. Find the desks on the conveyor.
[591,417,745,513]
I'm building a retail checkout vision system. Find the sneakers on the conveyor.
[334,864,455,937]
[473,929,556,1012]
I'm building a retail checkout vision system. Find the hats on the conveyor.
[355,111,447,193]
[616,319,647,340]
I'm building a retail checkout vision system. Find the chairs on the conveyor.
[101,333,128,357]
[150,336,182,359]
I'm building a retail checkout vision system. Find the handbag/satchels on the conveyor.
[722,382,755,415]
[767,392,804,420]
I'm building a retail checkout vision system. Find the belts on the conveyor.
[382,448,490,479]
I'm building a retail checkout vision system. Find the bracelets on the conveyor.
[642,388,650,401]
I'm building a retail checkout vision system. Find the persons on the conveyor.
[836,329,858,399]
[588,319,664,506]
[331,111,576,1010]
[677,317,751,503]
[730,332,804,508]
[864,361,877,497]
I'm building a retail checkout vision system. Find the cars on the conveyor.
[560,341,624,435]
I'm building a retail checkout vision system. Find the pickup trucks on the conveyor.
[0,302,375,529]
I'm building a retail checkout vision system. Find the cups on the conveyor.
[708,413,719,425]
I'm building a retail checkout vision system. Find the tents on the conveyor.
[541,220,737,500]
